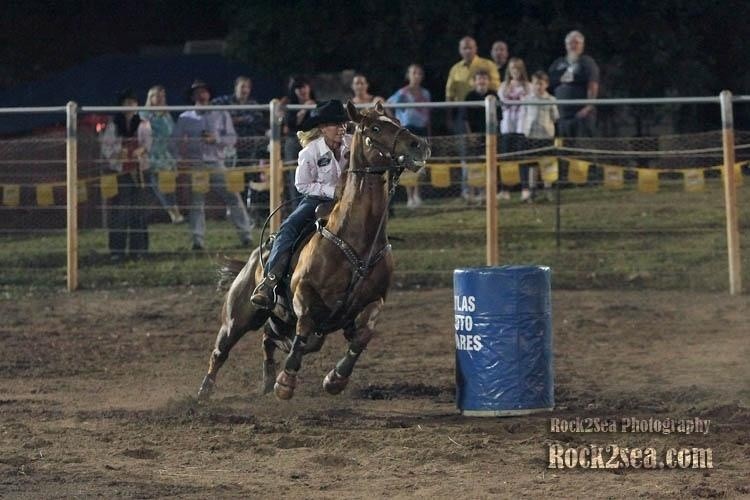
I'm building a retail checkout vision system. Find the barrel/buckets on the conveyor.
[453,265,557,417]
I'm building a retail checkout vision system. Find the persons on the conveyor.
[99,31,601,305]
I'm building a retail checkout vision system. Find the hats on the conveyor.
[115,86,142,101]
[185,78,217,101]
[301,97,352,132]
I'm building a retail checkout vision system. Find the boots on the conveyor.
[250,272,281,308]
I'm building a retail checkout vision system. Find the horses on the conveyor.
[195,96,433,406]
